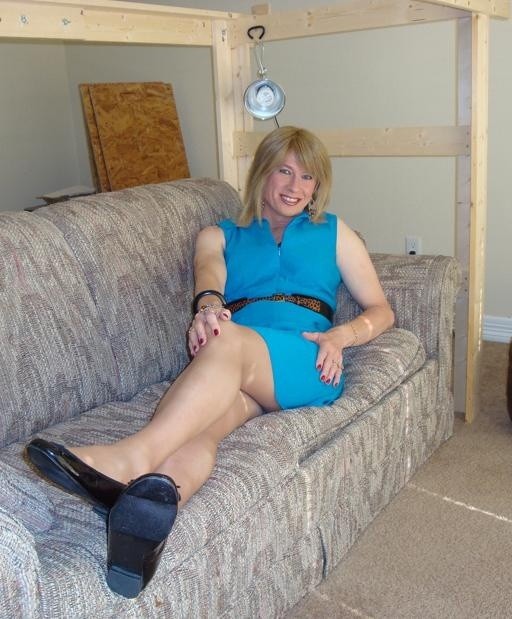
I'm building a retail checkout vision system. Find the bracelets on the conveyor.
[192,289,226,314]
[358,315,373,344]
[347,320,359,346]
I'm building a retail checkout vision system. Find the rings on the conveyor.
[333,360,339,369]
[188,327,195,334]
[199,304,223,315]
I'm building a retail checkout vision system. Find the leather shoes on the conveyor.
[26,438,179,599]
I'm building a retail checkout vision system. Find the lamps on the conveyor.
[242,25,286,122]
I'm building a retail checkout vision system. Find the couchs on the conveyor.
[0,183,461,619]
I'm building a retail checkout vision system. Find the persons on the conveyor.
[27,126,395,598]
